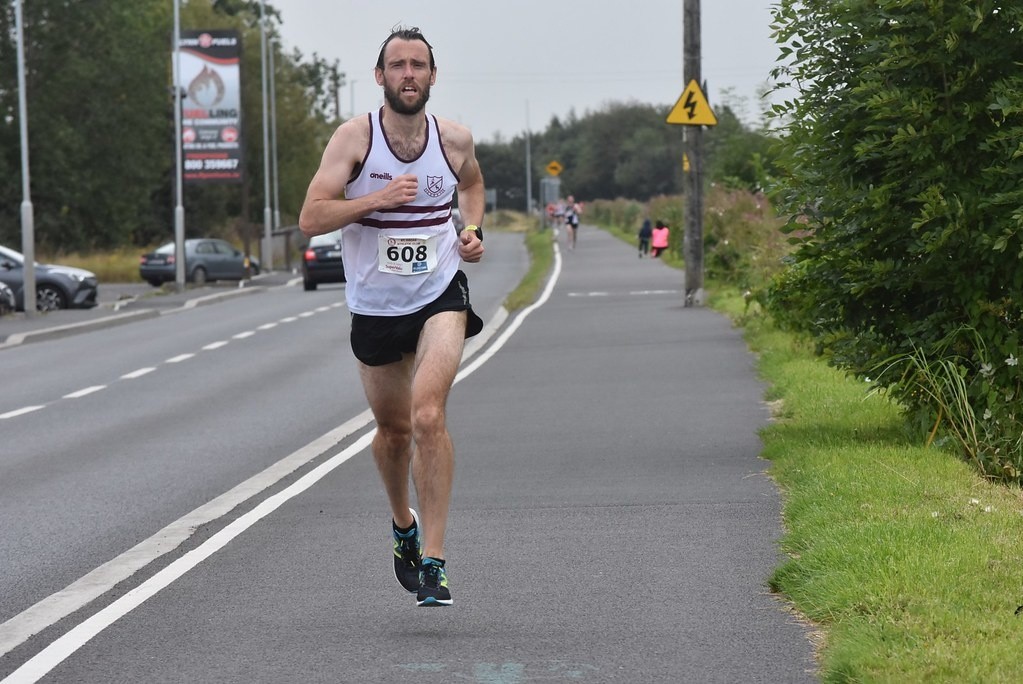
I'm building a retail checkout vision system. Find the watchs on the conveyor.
[465,225,483,242]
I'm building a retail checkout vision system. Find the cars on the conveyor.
[450,208,464,236]
[302,229,347,291]
[0,245,98,312]
[139,237,259,287]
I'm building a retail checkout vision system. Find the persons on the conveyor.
[638,219,653,259]
[649,221,669,258]
[299,27,485,608]
[548,198,565,228]
[565,195,581,250]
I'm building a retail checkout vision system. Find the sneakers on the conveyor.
[392,508,420,596]
[416,554,453,606]
